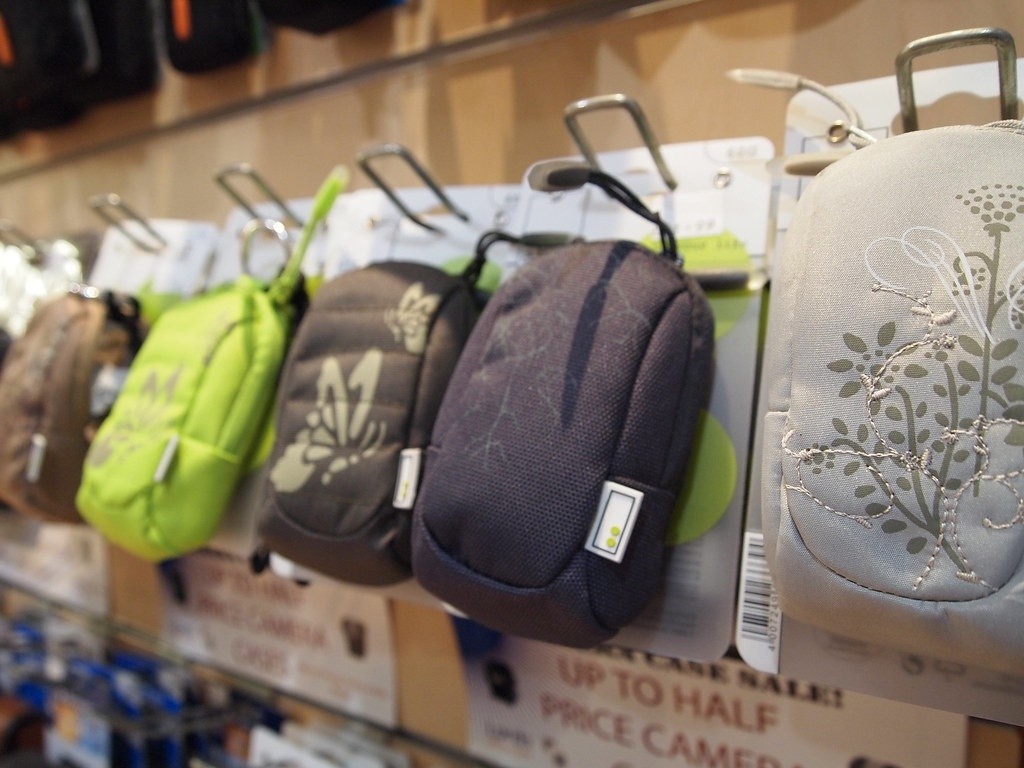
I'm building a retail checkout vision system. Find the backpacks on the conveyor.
[0,285,309,561]
[757,121,1024,670]
[407,167,718,651]
[252,243,497,587]
